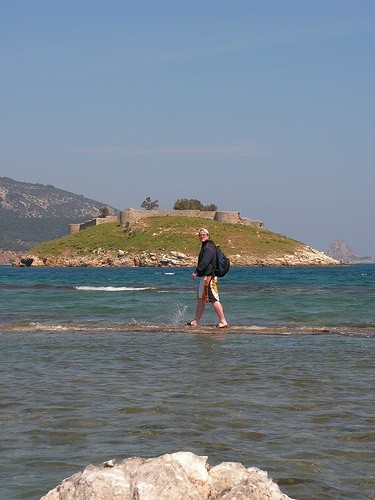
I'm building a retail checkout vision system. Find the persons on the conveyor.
[184,228,229,329]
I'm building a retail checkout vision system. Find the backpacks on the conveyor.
[207,240,230,277]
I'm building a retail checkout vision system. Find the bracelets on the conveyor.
[194,271,198,274]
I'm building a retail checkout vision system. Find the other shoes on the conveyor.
[216,320,228,328]
[186,320,200,326]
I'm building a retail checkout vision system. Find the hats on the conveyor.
[199,228,209,235]
